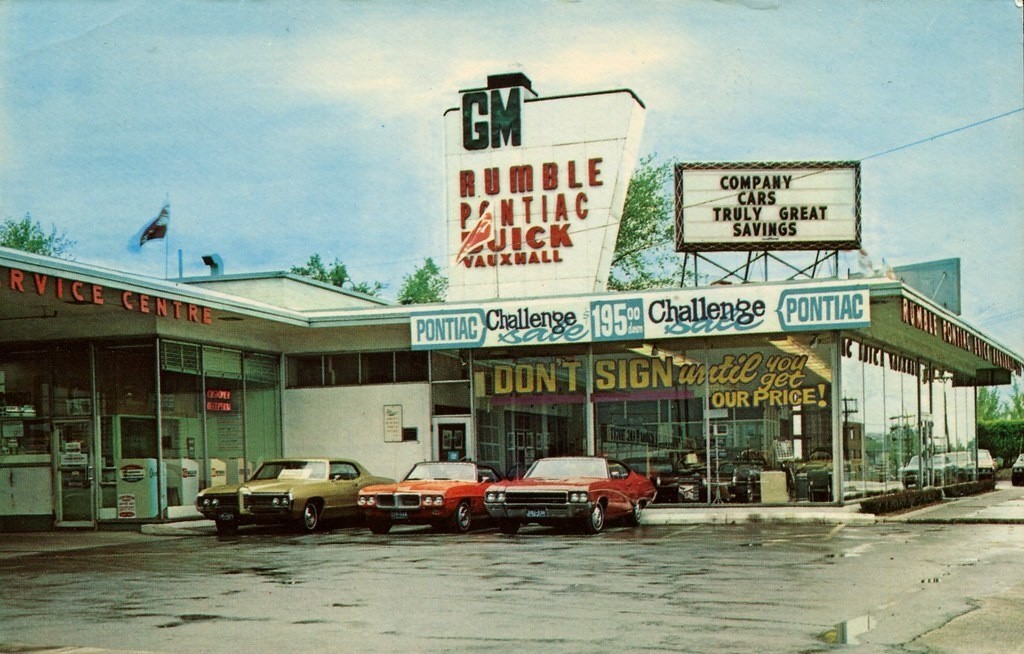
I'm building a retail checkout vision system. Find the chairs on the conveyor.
[805,470,831,501]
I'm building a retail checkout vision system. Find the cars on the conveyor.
[900,451,976,487]
[977,449,995,482]
[621,448,834,502]
[194,457,399,533]
[1011,454,1024,487]
[483,455,656,535]
[357,461,508,535]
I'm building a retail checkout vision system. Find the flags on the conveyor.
[456,203,495,265]
[139,204,169,246]
